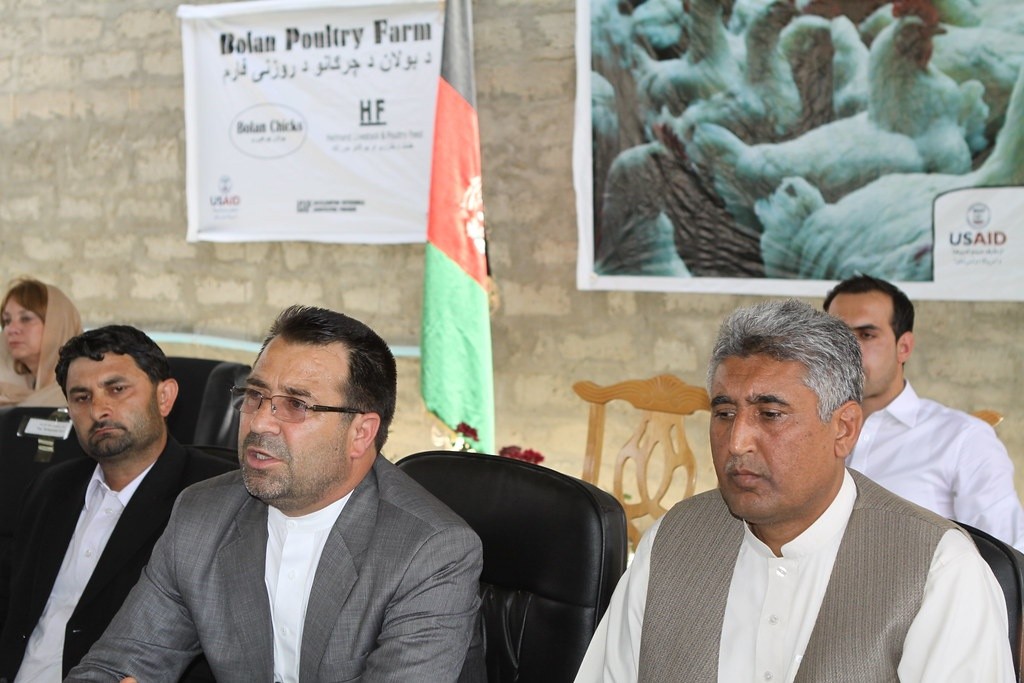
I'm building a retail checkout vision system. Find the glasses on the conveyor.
[230,386,366,424]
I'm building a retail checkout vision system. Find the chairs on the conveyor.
[165,356,252,449]
[392,450,628,683]
[571,373,710,553]
[947,518,1024,683]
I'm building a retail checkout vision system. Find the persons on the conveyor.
[821,274,1024,553]
[63,304,487,683]
[0,324,231,683]
[571,300,1020,683]
[1,276,83,408]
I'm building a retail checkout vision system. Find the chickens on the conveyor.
[590,0,1024,282]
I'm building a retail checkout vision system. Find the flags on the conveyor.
[417,76,497,457]
